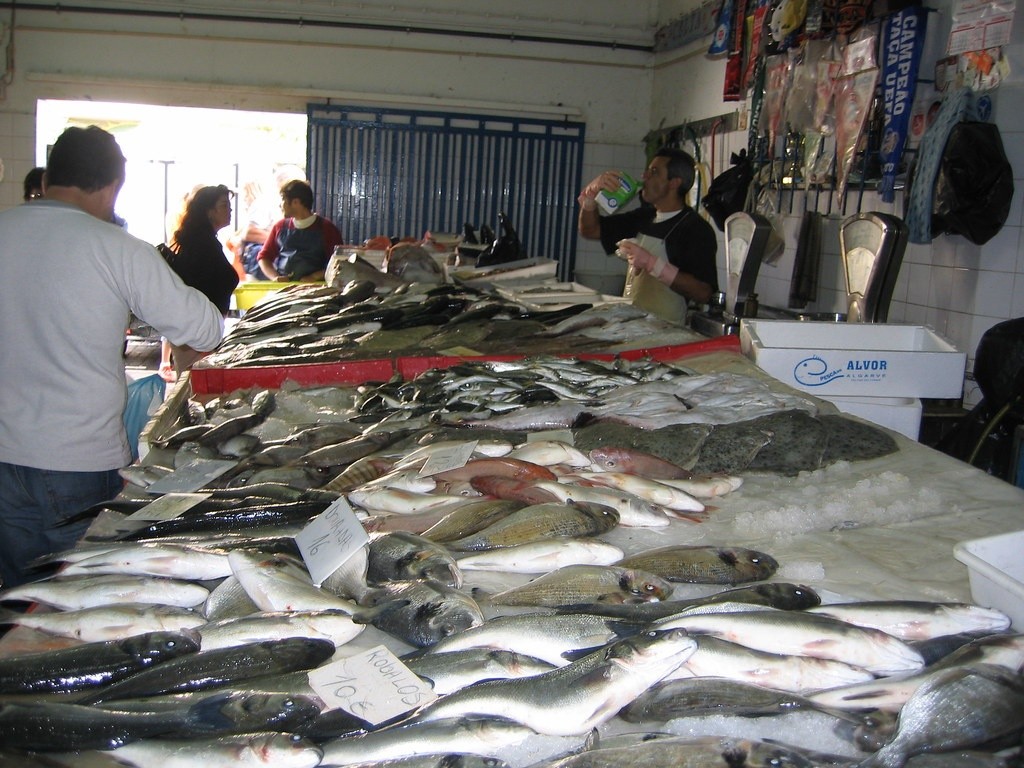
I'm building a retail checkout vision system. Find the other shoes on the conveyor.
[158,366,175,381]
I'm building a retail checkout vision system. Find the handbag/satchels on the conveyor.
[157,239,192,285]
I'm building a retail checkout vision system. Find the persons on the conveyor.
[23,164,343,380]
[0,126,225,595]
[578,147,719,325]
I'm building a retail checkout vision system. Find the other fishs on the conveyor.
[2,245,1023,767]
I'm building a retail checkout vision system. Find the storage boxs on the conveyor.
[448,254,602,307]
[741,318,966,399]
[952,532,1024,633]
[232,282,324,309]
[814,396,923,442]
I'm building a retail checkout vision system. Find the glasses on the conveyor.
[30,193,43,200]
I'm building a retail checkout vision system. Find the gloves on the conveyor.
[621,241,680,288]
[577,170,624,211]
